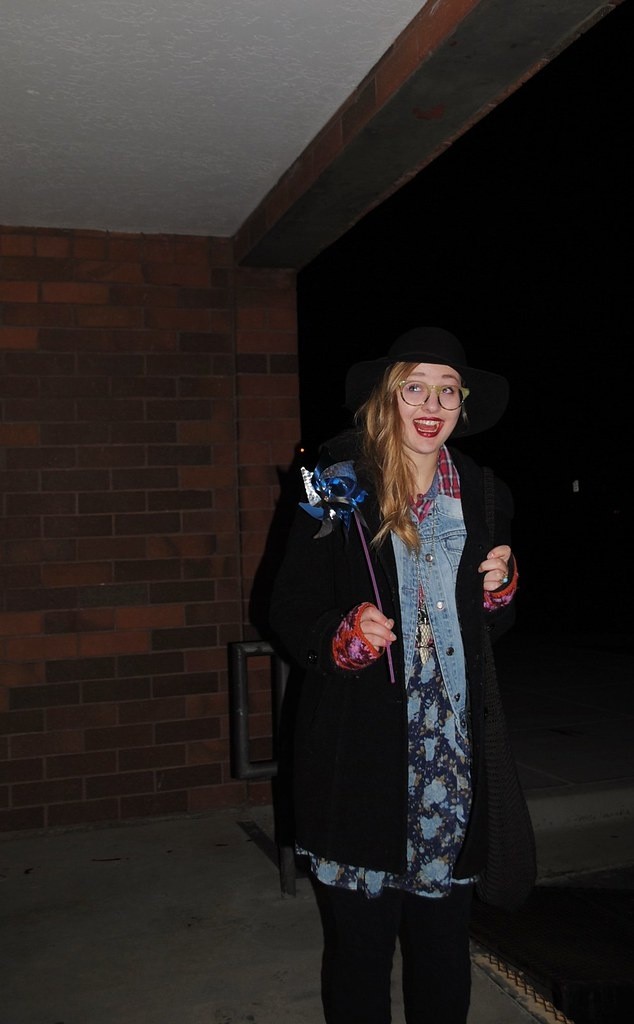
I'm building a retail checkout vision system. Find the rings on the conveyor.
[498,572,508,584]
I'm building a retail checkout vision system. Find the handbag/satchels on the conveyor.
[472,622,537,912]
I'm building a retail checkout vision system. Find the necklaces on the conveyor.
[415,452,441,663]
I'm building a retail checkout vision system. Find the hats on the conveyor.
[344,327,508,439]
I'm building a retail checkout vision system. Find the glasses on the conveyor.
[398,380,469,411]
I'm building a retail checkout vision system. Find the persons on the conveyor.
[249,324,520,1024]
